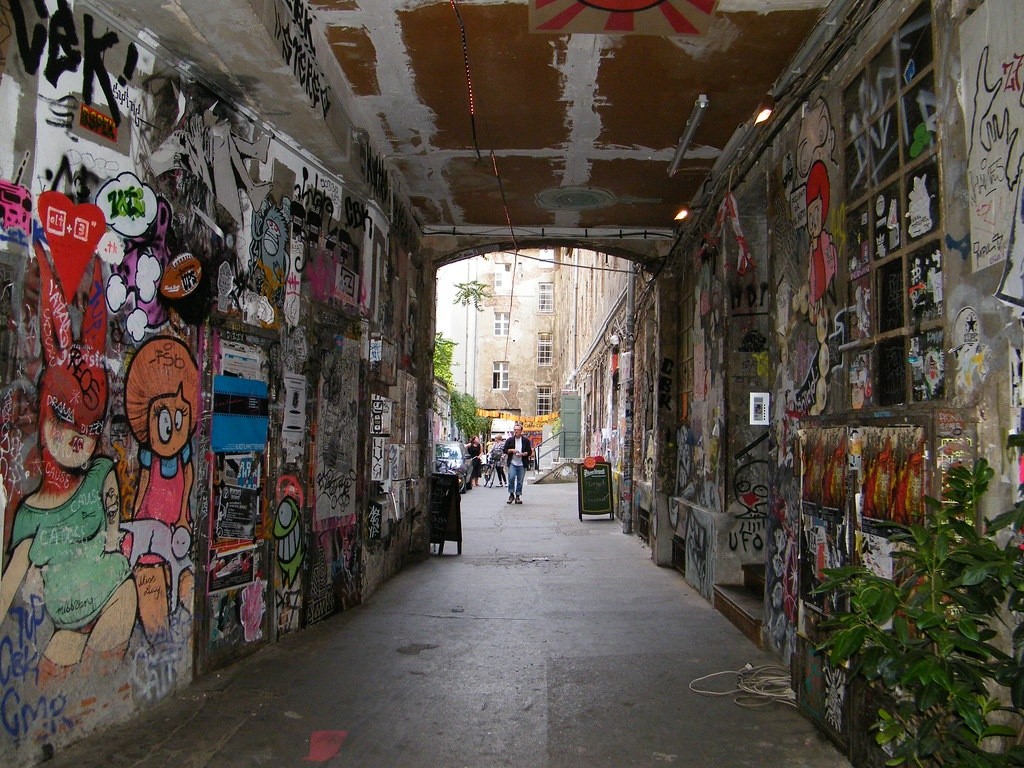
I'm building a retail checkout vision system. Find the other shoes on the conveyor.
[506,484,508,487]
[515,500,522,504]
[506,496,514,504]
[496,483,503,487]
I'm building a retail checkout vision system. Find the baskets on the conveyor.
[491,450,502,461]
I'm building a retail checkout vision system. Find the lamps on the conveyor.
[610,334,624,345]
[671,205,706,220]
[666,95,708,178]
[752,73,806,126]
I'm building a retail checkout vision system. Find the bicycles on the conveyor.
[481,447,504,489]
[434,458,466,493]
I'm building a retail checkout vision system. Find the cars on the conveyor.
[436,441,474,494]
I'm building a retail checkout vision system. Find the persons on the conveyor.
[502,424,532,504]
[466,436,483,487]
[486,434,509,487]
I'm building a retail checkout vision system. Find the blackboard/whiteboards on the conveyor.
[577,462,614,514]
[429,473,463,544]
